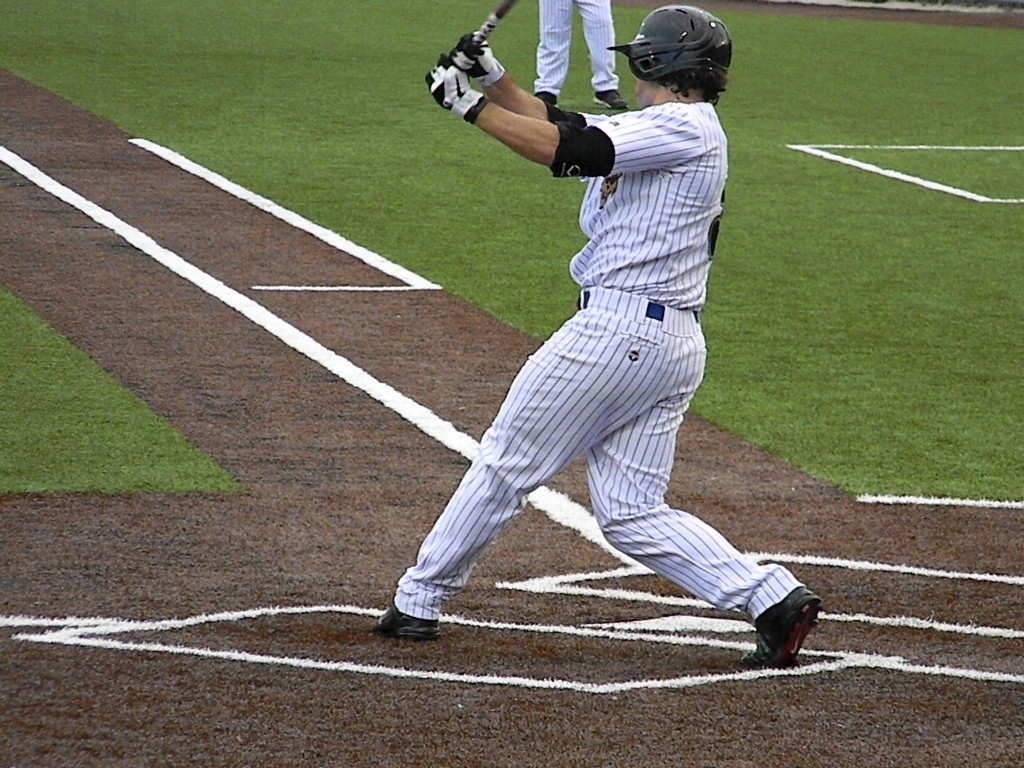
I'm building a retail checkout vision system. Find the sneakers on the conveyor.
[372,606,440,642]
[593,88,627,108]
[740,588,821,668]
[536,92,557,109]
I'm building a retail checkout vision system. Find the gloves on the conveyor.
[424,52,489,124]
[449,30,505,88]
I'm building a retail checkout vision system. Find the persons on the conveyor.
[370,8,822,667]
[530,0,629,108]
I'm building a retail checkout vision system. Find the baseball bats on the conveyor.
[472,0,518,52]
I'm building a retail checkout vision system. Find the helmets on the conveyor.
[606,5,733,90]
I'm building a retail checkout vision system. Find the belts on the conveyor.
[584,291,699,323]
